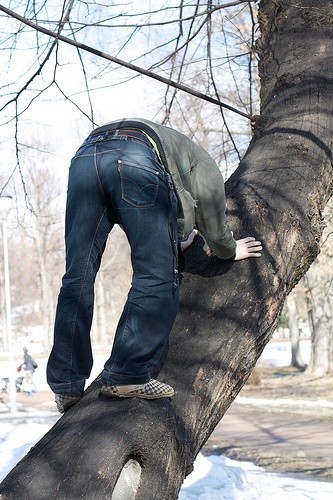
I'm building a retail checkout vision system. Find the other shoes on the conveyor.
[54,394,80,414]
[101,379,174,399]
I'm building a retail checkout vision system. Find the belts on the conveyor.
[87,130,154,149]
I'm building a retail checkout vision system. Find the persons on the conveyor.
[46,116,262,413]
[22,346,35,391]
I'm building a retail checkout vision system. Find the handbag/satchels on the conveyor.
[32,361,38,368]
